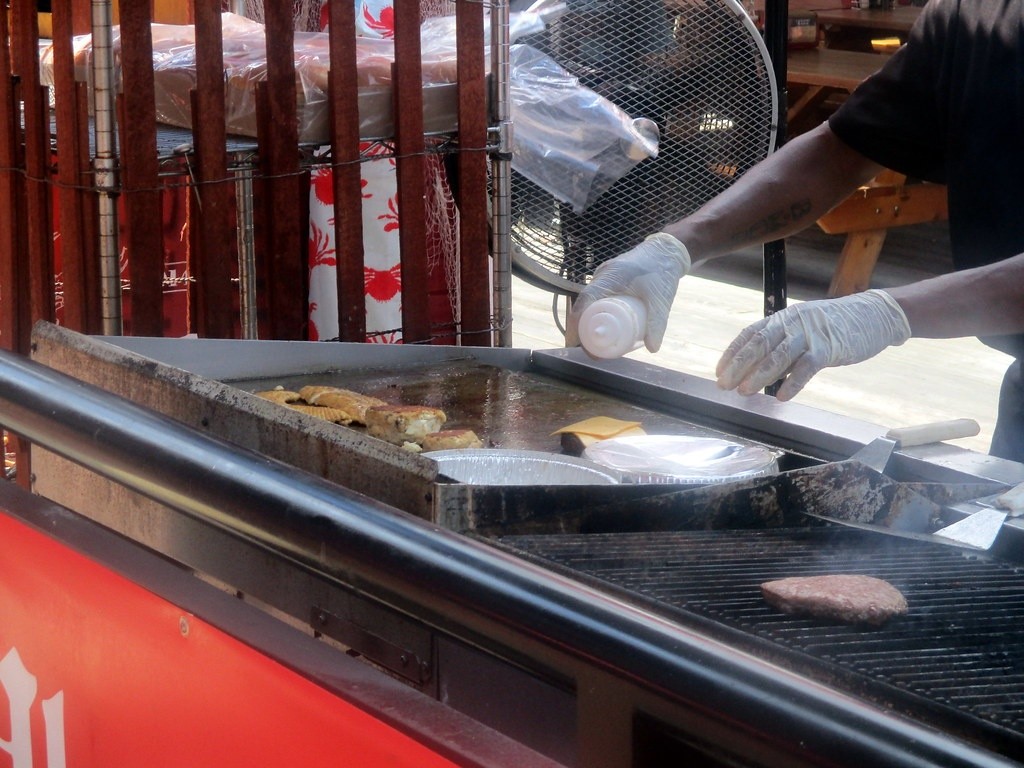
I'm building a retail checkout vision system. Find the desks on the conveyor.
[786,0,929,32]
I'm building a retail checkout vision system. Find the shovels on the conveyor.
[842,417,981,474]
[803,492,1024,553]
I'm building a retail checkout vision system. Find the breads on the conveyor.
[33,13,459,145]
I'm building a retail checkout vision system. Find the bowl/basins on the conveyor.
[584,436,779,485]
[419,449,621,488]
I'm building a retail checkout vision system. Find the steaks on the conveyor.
[760,575,908,625]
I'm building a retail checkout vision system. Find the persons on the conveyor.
[563,0,1024,459]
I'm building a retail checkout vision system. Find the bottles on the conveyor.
[577,293,647,361]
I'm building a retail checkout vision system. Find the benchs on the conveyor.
[783,45,959,295]
